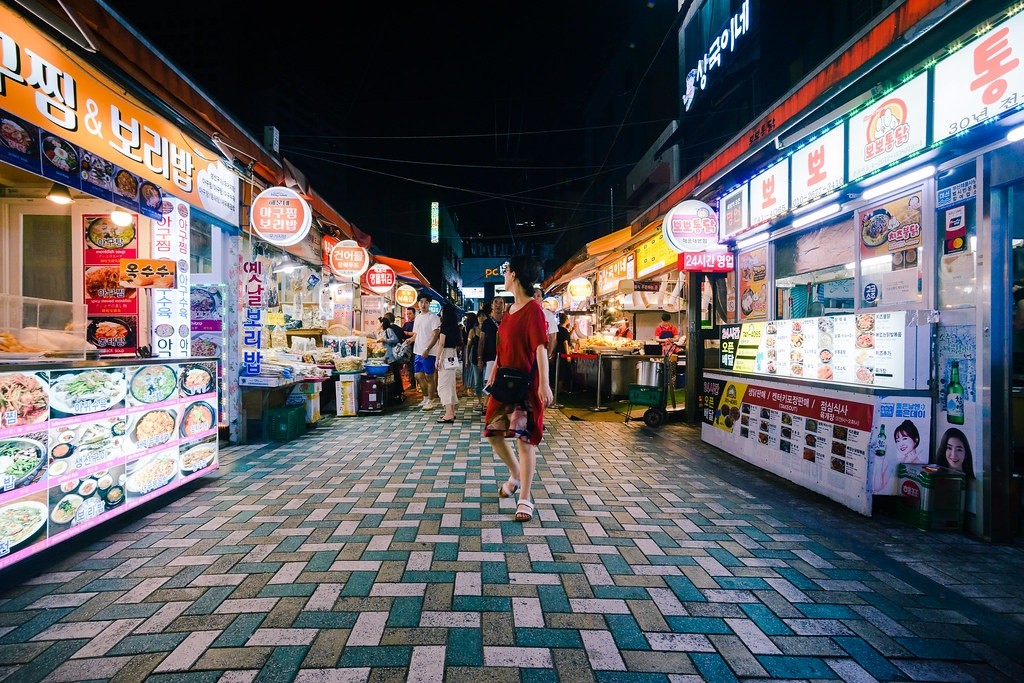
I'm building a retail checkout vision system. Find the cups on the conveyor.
[118,472,126,485]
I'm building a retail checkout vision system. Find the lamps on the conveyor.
[272,246,306,274]
[45,182,73,204]
[312,264,338,284]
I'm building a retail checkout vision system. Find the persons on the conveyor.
[352,288,576,424]
[874,420,924,492]
[937,428,976,479]
[483,254,554,522]
[615,320,633,339]
[654,313,679,344]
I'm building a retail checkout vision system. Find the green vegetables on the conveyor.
[0,443,41,486]
[58,500,72,513]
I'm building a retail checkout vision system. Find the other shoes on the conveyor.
[482,407,487,416]
[437,415,456,423]
[475,403,484,411]
[417,400,434,411]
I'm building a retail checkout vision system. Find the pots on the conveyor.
[636,361,664,391]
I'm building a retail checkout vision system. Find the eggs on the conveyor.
[82,171,89,180]
[54,147,68,160]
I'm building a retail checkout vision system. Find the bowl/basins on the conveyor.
[0,438,47,492]
[48,400,217,475]
[102,485,125,506]
[597,345,633,355]
[365,363,391,375]
[181,365,213,396]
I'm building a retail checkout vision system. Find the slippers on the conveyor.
[514,499,535,522]
[499,475,521,499]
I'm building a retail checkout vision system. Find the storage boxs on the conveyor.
[262,373,362,443]
[896,462,967,531]
[628,384,662,406]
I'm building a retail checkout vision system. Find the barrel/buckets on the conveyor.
[358,376,383,411]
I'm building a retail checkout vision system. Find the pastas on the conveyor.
[0,373,48,430]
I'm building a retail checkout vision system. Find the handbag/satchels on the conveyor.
[391,343,405,361]
[489,365,534,404]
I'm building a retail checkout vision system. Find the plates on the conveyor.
[51,469,126,526]
[861,209,893,247]
[126,455,179,493]
[49,375,126,413]
[892,250,916,266]
[0,501,49,548]
[28,374,49,422]
[49,460,67,476]
[765,311,874,384]
[130,364,177,403]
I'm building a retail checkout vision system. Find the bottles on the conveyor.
[876,424,888,456]
[578,344,594,354]
[573,337,579,353]
[946,361,964,425]
